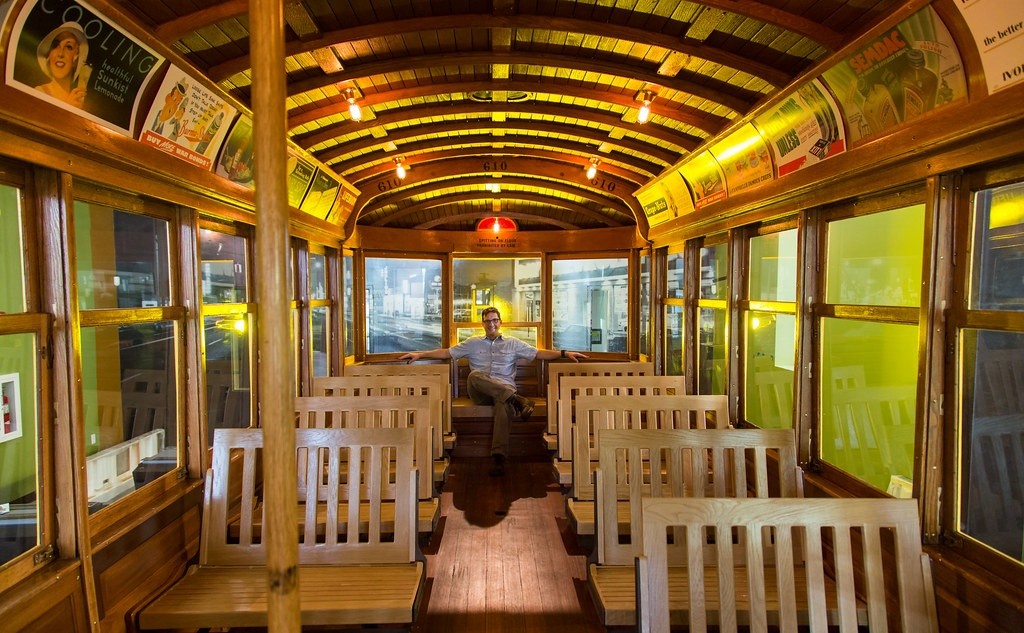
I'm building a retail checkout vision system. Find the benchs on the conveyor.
[565,394,735,535]
[343,364,456,450]
[303,375,450,482]
[671,345,1024,561]
[230,394,441,541]
[589,427,807,626]
[80,359,251,477]
[552,374,687,484]
[124,425,429,632]
[542,361,654,448]
[363,356,631,419]
[635,496,940,632]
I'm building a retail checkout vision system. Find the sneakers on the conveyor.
[511,395,535,419]
[490,453,505,475]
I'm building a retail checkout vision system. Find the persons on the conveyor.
[397,307,590,475]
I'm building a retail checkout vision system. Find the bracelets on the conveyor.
[561,350,567,358]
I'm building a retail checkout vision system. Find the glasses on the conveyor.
[483,318,499,324]
